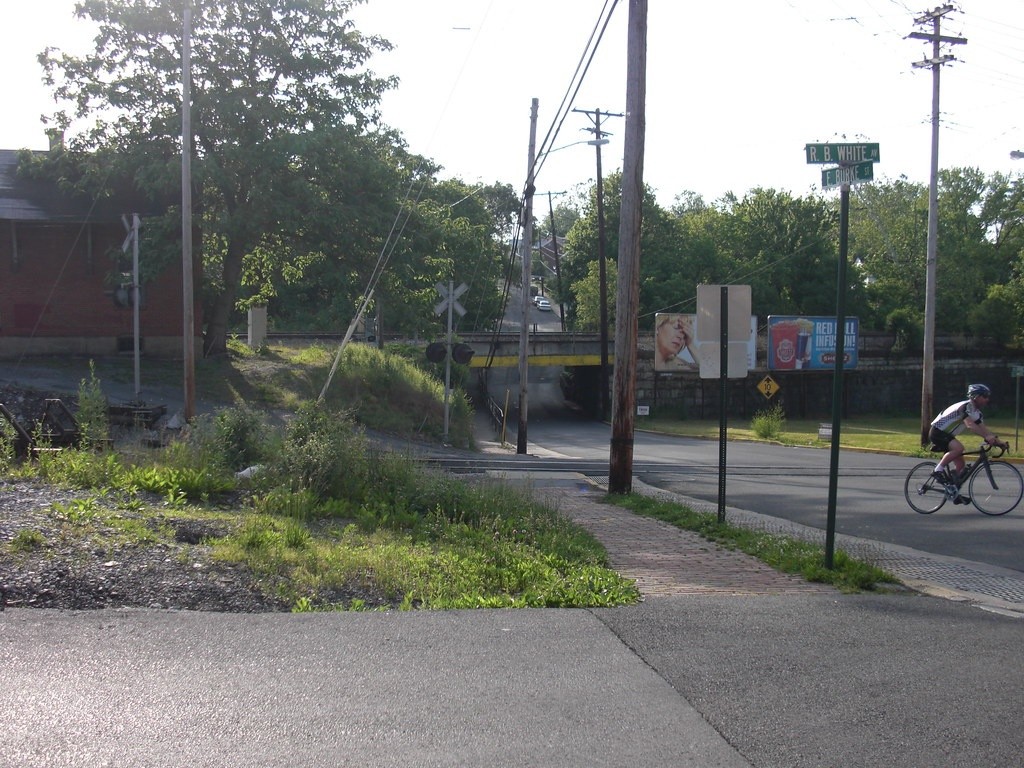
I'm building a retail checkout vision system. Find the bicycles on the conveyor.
[903,435,1024,516]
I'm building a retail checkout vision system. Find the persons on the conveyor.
[930,384,1010,506]
[656,314,700,369]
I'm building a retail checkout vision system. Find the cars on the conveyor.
[532,296,545,306]
[537,300,551,311]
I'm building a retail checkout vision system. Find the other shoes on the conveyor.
[929,469,947,484]
[954,495,971,505]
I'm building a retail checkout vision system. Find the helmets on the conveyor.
[967,383,991,400]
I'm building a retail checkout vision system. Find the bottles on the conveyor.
[235,464,264,480]
[956,462,971,483]
[950,468,958,485]
